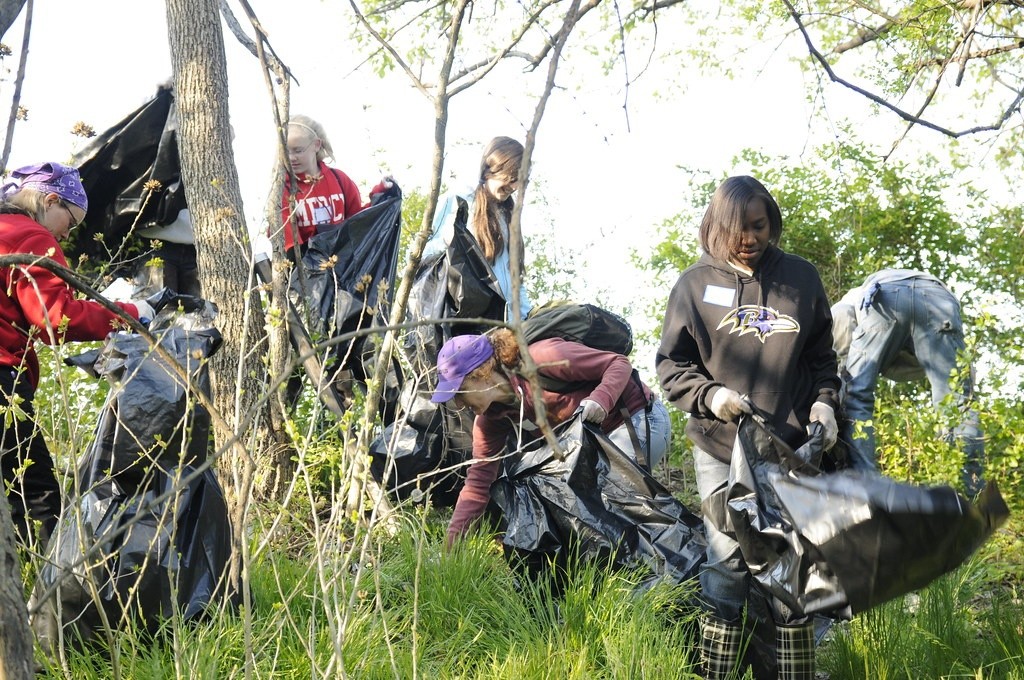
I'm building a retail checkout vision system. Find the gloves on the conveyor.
[580,400,605,424]
[135,300,156,323]
[860,282,880,310]
[809,400,839,453]
[710,388,752,422]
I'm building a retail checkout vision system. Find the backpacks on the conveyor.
[512,304,634,393]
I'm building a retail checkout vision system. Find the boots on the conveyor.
[774,618,816,680]
[698,611,742,680]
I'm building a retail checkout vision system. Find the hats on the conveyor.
[432,335,493,403]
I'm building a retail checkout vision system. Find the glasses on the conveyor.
[287,141,312,157]
[60,202,78,230]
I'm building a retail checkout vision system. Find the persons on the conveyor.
[431,327,672,562]
[265,115,395,272]
[830,267,986,496]
[0,160,159,559]
[151,75,236,298]
[407,136,532,325]
[655,175,843,680]
